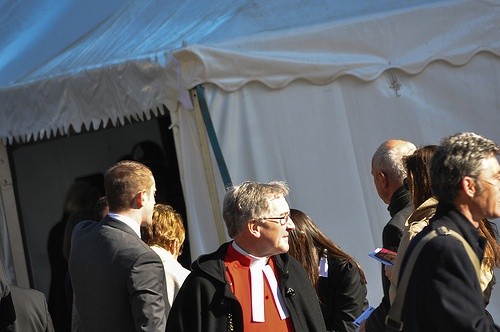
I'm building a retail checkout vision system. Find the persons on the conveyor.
[353,133,500,332]
[165,181,326,332]
[286,209,368,332]
[0,156,192,332]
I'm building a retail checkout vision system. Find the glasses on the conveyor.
[258,213,292,225]
[178,244,184,256]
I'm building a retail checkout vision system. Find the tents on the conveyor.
[0,0,500,327]
